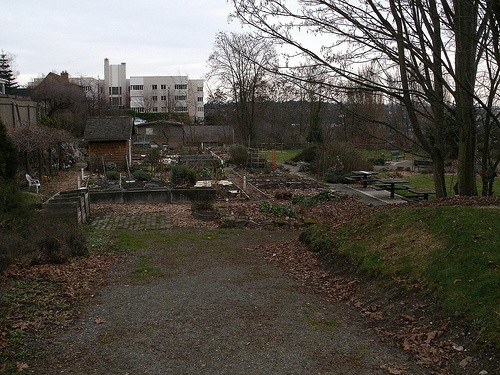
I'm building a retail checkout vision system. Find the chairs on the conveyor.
[26,174,40,194]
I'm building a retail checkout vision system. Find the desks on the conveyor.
[346,170,436,205]
